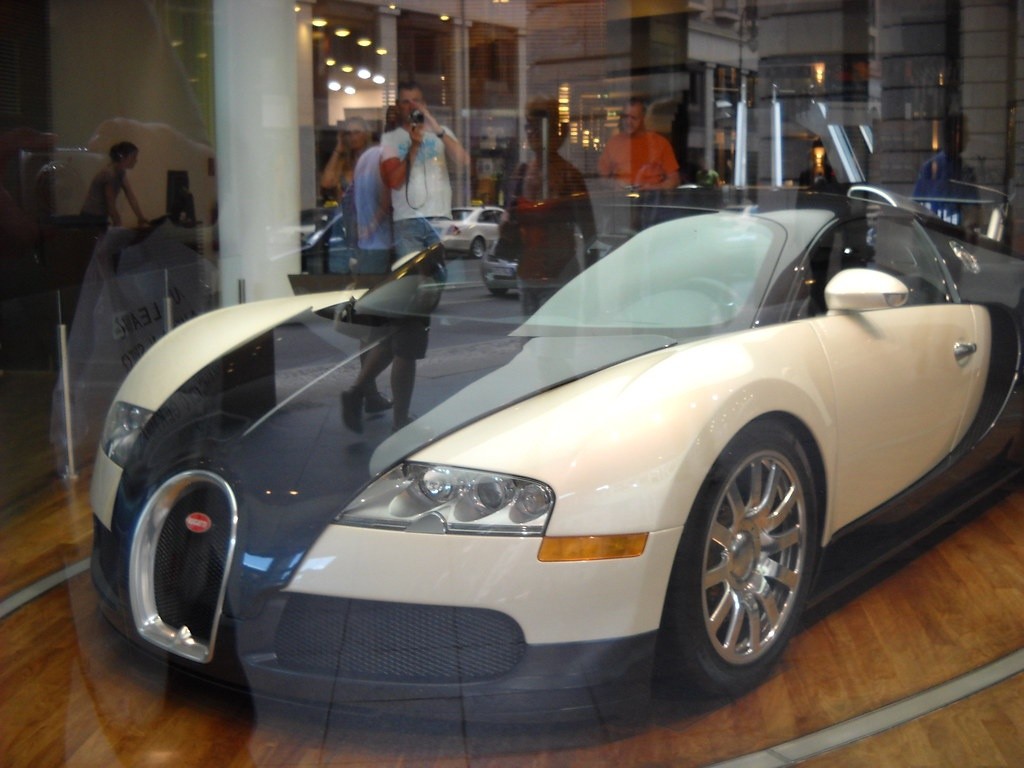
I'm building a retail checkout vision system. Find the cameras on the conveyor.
[410,109,425,123]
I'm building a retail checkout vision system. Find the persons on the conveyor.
[378,83,471,250]
[80,141,153,232]
[499,99,598,320]
[320,115,373,249]
[341,117,439,435]
[911,112,980,304]
[0,1,258,768]
[598,99,681,190]
[796,140,846,318]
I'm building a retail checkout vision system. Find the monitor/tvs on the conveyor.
[166,169,196,227]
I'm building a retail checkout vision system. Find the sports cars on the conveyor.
[87,190,1024,717]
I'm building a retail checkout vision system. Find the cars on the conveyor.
[281,206,357,277]
[427,207,516,258]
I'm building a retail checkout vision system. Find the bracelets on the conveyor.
[436,128,445,138]
[333,149,341,157]
